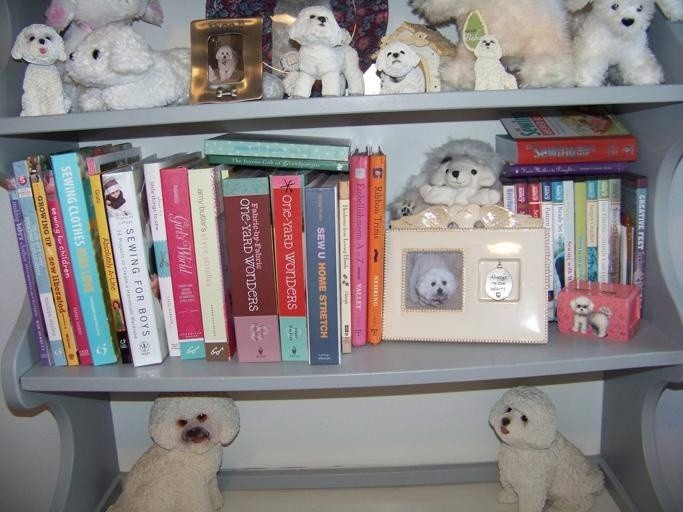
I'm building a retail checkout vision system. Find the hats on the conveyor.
[103,178,122,195]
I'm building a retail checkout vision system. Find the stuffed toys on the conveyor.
[109,392,240,510]
[9,24,69,117]
[287,4,362,100]
[47,1,163,114]
[385,139,502,222]
[565,0,683,86]
[489,385,604,512]
[474,33,518,91]
[411,1,586,90]
[375,42,424,95]
[64,20,191,109]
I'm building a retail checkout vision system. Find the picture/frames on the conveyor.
[187,17,263,102]
[384,206,550,344]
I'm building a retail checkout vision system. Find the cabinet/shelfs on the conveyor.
[0,1,680,504]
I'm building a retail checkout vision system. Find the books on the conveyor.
[339,173,352,353]
[551,179,564,322]
[575,182,588,289]
[543,181,555,321]
[50,142,132,366]
[270,168,313,362]
[206,130,352,161]
[222,170,281,363]
[621,211,633,285]
[100,154,170,367]
[504,162,638,176]
[349,147,369,347]
[159,155,206,360]
[530,180,542,217]
[89,164,132,363]
[143,151,187,357]
[87,147,142,175]
[208,155,348,171]
[304,172,341,365]
[496,106,631,138]
[621,172,648,319]
[369,144,386,344]
[495,134,637,165]
[4,175,51,368]
[563,180,575,290]
[221,166,236,179]
[188,157,237,361]
[609,178,620,283]
[30,173,79,366]
[504,182,517,214]
[517,181,530,214]
[12,153,68,367]
[587,180,598,282]
[41,169,93,366]
[598,179,609,284]
